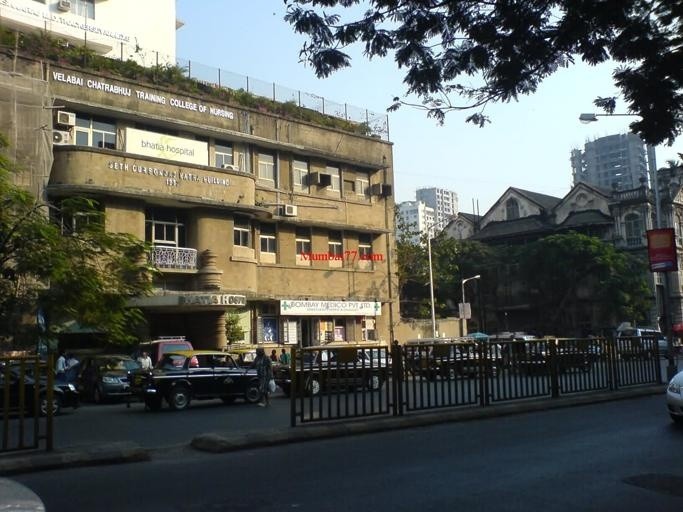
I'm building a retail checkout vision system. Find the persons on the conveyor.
[54,350,67,373]
[279,348,289,365]
[269,349,278,362]
[65,353,79,369]
[243,346,273,409]
[136,350,153,371]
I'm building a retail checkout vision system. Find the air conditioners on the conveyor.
[57,0,72,11]
[284,204,298,217]
[221,164,239,171]
[52,109,76,146]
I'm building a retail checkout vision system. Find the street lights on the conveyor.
[425,213,458,337]
[580,113,679,380]
[462,274,481,337]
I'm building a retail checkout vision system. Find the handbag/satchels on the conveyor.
[268,379,276,393]
